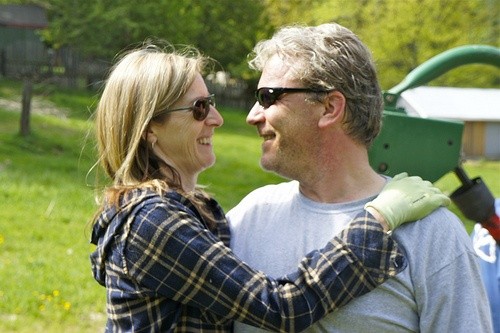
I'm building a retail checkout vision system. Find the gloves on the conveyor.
[364,172,449,231]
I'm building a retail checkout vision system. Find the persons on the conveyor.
[88,45,453,333]
[225,23,493,333]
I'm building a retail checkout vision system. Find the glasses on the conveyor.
[155,94,216,121]
[255,87,332,108]
